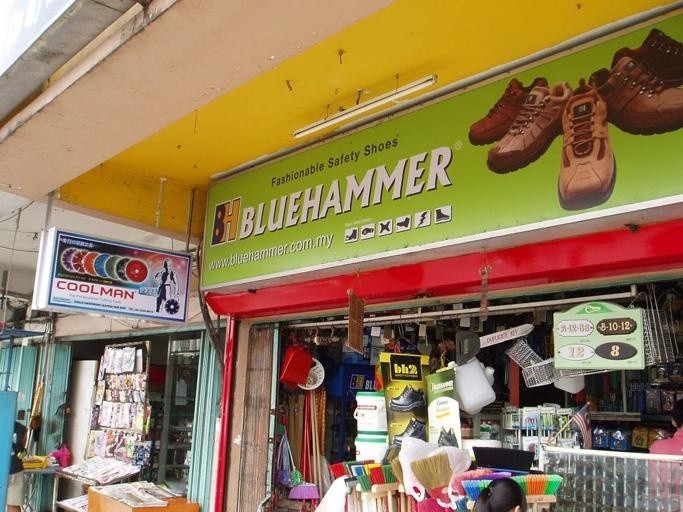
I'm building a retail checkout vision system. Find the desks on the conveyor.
[87,479,200,511]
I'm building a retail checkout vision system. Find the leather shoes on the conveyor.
[438,426,459,447]
[393,419,426,447]
[389,385,425,412]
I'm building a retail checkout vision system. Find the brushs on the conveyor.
[275,413,302,486]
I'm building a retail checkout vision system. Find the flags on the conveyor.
[573,405,593,450]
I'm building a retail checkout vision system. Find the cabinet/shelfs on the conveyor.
[563,324,683,455]
[144,365,197,482]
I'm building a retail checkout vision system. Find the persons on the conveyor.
[647,400,683,512]
[5,420,28,512]
[472,476,528,512]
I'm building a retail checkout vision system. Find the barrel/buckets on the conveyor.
[354,436,391,468]
[353,390,389,441]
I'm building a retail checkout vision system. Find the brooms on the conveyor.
[333,454,563,511]
[288,393,321,500]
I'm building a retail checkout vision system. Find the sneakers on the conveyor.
[468,28,683,211]
